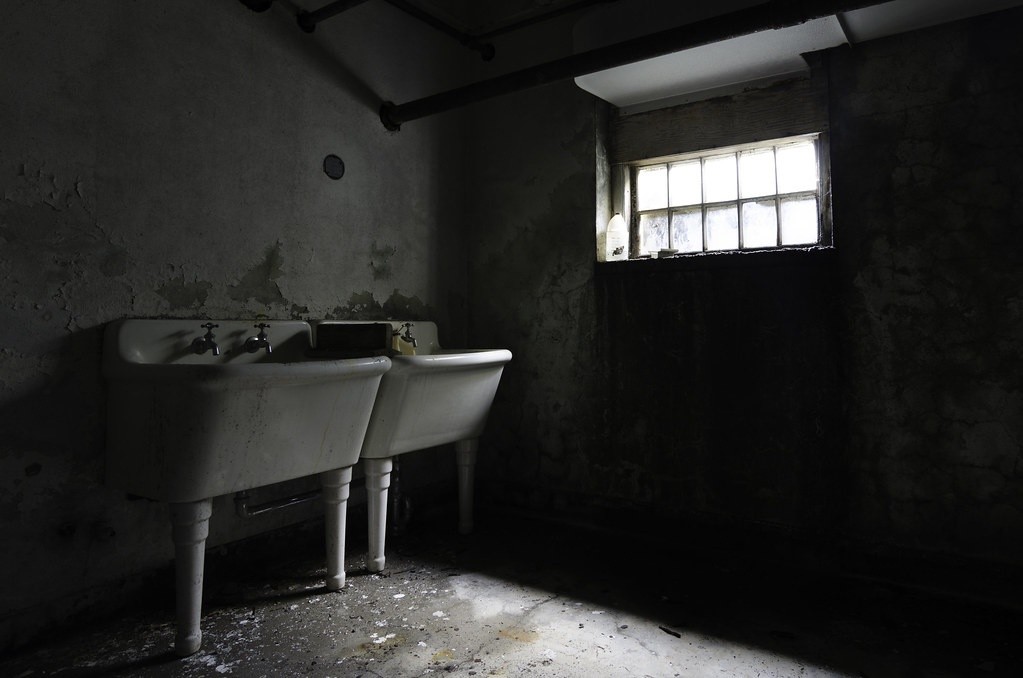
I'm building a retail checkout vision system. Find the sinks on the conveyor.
[314,319,513,459]
[101,318,393,505]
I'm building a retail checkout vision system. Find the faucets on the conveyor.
[191,322,221,357]
[243,322,273,354]
[400,322,418,348]
